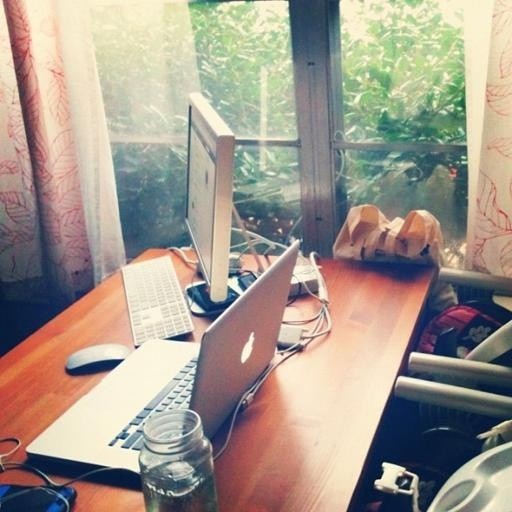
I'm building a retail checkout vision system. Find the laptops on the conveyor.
[26,239,300,474]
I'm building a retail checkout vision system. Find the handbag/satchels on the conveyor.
[330,203,446,267]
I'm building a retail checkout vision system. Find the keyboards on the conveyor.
[121,255,194,347]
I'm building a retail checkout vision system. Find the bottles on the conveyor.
[138,408,219,512]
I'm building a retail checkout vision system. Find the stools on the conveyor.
[374,266,512,512]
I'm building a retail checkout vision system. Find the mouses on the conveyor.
[65,344,131,373]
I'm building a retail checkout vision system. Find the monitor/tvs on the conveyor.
[185,91,250,316]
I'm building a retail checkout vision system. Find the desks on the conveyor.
[0,246,439,512]
